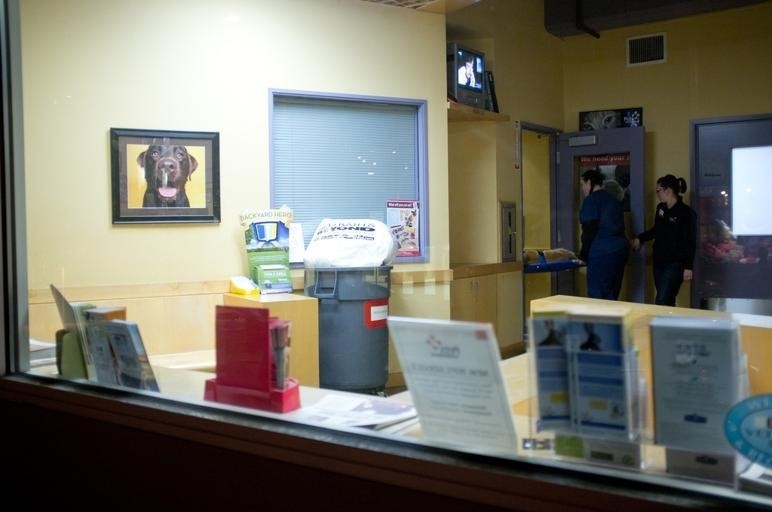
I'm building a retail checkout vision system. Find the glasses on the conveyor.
[655,188,662,192]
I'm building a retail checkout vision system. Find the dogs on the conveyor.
[135,143,199,208]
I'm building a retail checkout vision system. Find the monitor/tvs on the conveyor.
[447,43,486,110]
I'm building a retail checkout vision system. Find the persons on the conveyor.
[578,166,698,307]
[457,53,476,88]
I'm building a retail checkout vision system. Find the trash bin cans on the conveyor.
[314,267,393,391]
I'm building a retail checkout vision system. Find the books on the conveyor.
[49,280,161,393]
[386,200,420,257]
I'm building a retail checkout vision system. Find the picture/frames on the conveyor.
[106,127,221,223]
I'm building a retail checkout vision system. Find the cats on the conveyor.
[581,109,623,131]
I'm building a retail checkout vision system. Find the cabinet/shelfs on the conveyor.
[224,291,320,387]
[450,274,497,340]
[496,270,523,350]
